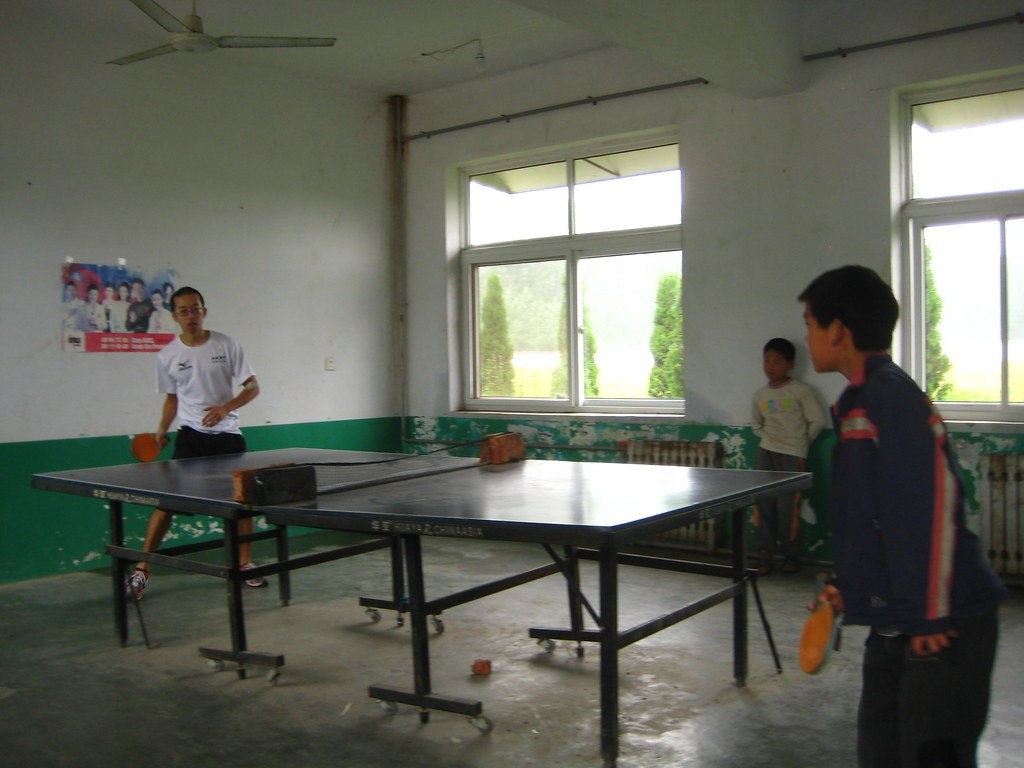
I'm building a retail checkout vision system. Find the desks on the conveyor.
[30,446,815,768]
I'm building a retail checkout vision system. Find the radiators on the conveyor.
[623,439,724,554]
[977,452,1024,581]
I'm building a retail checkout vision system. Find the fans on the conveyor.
[104,0,338,67]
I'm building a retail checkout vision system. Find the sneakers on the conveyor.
[124,568,150,601]
[240,561,268,587]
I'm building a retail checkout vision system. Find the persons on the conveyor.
[751,338,826,572]
[124,287,260,599]
[800,266,998,768]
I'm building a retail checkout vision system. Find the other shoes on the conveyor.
[754,559,774,576]
[781,557,798,572]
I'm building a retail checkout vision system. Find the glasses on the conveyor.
[173,306,206,317]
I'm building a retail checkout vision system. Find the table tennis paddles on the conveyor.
[798,599,842,676]
[133,430,157,463]
